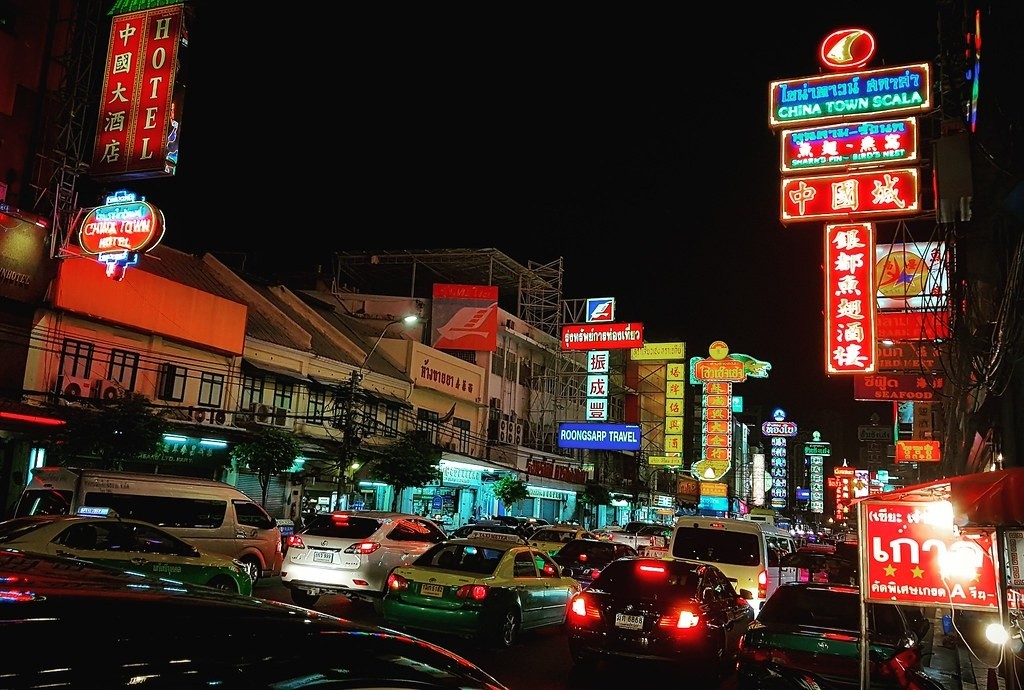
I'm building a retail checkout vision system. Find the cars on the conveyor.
[0,514,253,596]
[0,549,508,690]
[456,514,859,594]
[735,582,930,690]
[562,557,755,676]
[381,532,580,647]
[278,512,455,609]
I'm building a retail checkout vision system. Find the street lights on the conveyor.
[334,314,419,508]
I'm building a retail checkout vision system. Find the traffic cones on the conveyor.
[984,667,999,690]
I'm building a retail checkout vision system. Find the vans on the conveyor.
[14,464,283,586]
[666,512,800,621]
